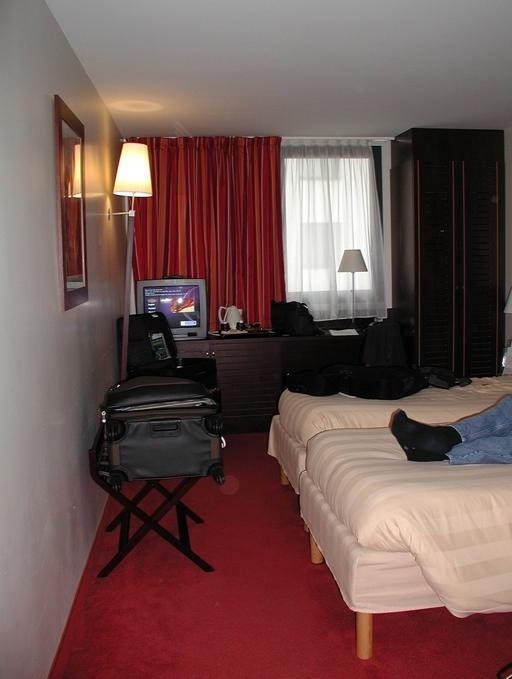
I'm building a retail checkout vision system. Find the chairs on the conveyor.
[116,314,226,452]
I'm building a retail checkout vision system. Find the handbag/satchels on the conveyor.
[284,359,341,395]
[413,367,456,390]
[271,300,324,336]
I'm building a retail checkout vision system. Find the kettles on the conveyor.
[218,306,243,332]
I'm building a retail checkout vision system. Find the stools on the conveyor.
[89,429,216,577]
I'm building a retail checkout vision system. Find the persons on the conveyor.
[389,394,512,463]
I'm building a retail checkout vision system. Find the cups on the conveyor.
[221,322,243,331]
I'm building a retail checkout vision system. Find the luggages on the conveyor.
[100,376,226,492]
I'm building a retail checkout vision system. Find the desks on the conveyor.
[175,328,365,435]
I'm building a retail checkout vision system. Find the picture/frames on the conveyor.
[56,93,89,312]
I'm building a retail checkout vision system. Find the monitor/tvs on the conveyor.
[136,279,208,338]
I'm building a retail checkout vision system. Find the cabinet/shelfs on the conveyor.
[393,127,505,380]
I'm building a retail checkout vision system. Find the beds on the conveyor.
[267,375,512,534]
[300,423,512,660]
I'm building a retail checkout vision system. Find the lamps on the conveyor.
[338,250,368,329]
[107,143,152,217]
